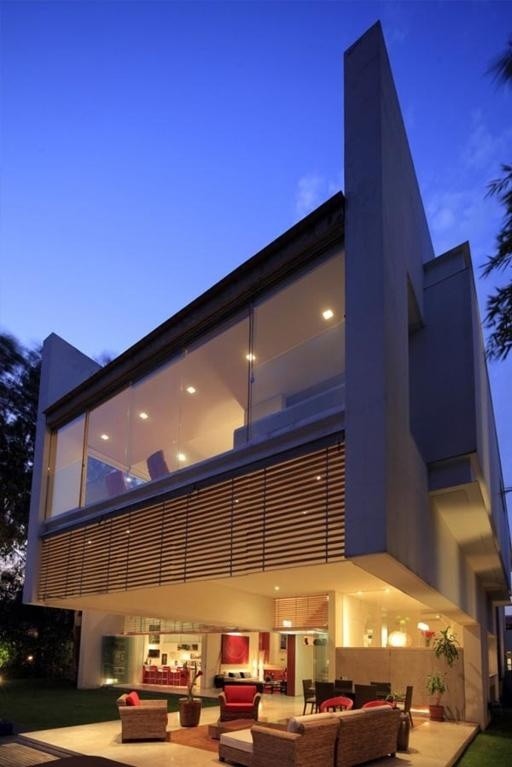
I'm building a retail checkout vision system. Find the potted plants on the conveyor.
[178,662,203,728]
[426,672,449,722]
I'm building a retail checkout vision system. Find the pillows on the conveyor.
[230,672,252,678]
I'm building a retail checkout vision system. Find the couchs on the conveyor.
[219,705,400,767]
[215,673,258,688]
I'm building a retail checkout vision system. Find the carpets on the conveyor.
[166,722,277,755]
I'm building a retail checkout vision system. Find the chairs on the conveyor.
[302,678,415,728]
[218,685,261,723]
[116,693,168,742]
[105,471,126,497]
[146,449,169,480]
[143,666,179,685]
[208,719,255,740]
